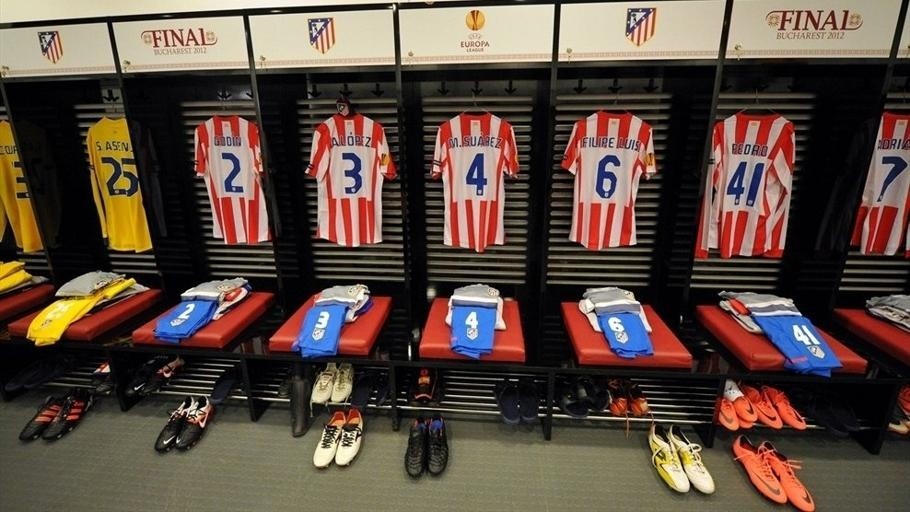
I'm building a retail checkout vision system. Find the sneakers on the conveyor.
[129,353,184,398]
[718,378,809,431]
[153,394,213,455]
[91,359,115,394]
[312,409,364,468]
[279,368,291,398]
[734,434,819,512]
[607,377,650,419]
[406,413,449,478]
[310,362,356,404]
[647,422,716,495]
[407,366,446,408]
[19,387,93,443]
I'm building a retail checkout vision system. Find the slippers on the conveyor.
[494,377,539,422]
[351,370,387,409]
[555,375,609,418]
[209,364,241,403]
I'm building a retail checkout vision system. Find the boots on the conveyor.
[290,368,308,437]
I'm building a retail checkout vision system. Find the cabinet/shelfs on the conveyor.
[1,1,909,454]
